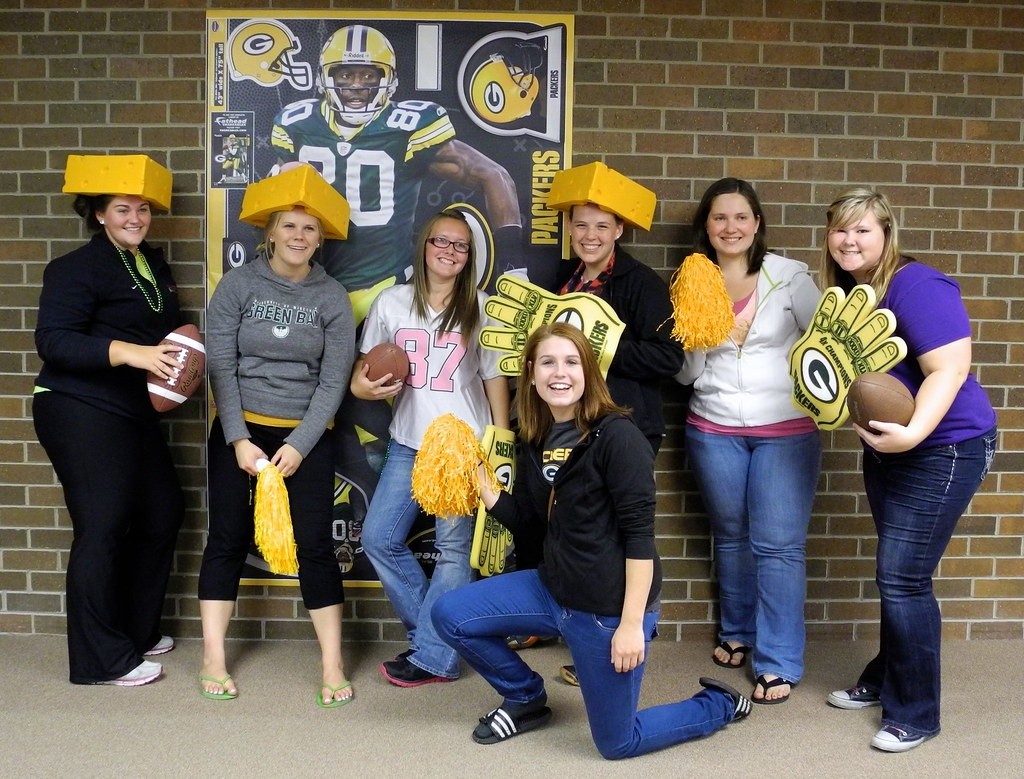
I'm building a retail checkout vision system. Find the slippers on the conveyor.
[713,641,748,668]
[317,681,354,708]
[472,705,552,744]
[751,674,791,704]
[699,676,753,721]
[199,674,238,700]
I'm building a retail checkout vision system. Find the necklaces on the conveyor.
[115,242,162,312]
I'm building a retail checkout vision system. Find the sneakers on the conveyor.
[827,683,883,709]
[394,649,417,662]
[870,723,933,753]
[143,635,174,655]
[380,659,456,688]
[86,660,163,687]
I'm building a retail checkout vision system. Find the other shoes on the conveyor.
[560,665,580,687]
[505,632,537,649]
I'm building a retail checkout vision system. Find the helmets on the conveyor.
[315,24,398,129]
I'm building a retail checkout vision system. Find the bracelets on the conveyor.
[473,461,483,470]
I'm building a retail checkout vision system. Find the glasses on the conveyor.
[425,237,470,254]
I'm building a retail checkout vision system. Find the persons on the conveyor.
[199,164,356,708]
[266,23,526,530]
[545,160,684,687]
[819,188,997,752]
[32,152,187,687]
[350,210,516,688]
[431,323,753,760]
[675,177,827,705]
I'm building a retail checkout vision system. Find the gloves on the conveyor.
[788,284,908,430]
[477,276,626,384]
[469,425,516,576]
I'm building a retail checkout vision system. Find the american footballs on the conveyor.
[361,342,410,389]
[848,371,916,436]
[146,322,207,414]
